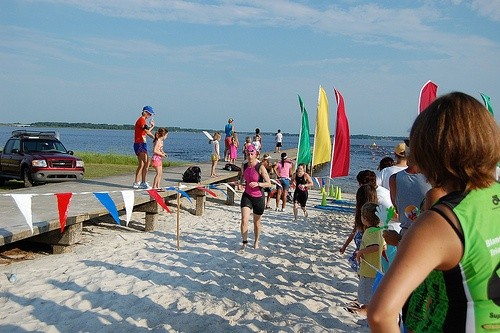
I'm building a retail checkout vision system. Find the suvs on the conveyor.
[0,126,86,189]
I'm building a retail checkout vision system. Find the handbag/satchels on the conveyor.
[224,164,240,172]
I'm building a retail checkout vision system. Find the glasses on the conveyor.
[146,111,152,116]
[246,149,254,152]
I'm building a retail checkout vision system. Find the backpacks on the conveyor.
[183,166,202,183]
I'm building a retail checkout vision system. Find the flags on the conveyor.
[329,87,350,178]
[312,84,331,165]
[417,80,438,115]
[295,93,311,168]
[480,92,495,117]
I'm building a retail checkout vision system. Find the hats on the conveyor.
[265,156,272,159]
[396,143,405,157]
[246,145,256,155]
[143,105,156,115]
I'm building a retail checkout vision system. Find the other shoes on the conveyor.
[140,183,150,189]
[281,208,285,212]
[347,301,369,315]
[354,319,369,326]
[275,208,279,211]
[132,182,147,189]
[265,206,272,209]
[157,188,165,192]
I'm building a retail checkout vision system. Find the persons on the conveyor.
[208,132,221,178]
[133,105,155,188]
[341,90,500,333]
[291,164,314,221]
[150,127,168,189]
[223,118,234,161]
[224,128,271,210]
[236,144,271,254]
[273,152,292,212]
[273,129,282,154]
